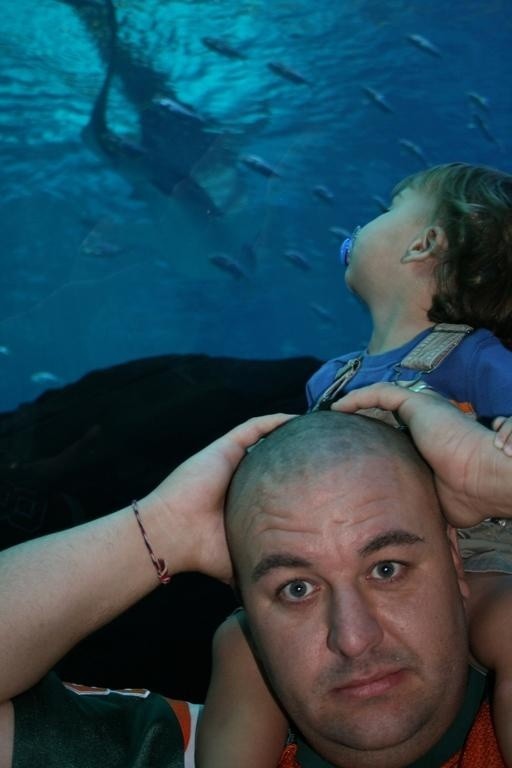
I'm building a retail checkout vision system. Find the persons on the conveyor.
[1,380,509,767]
[191,156,509,767]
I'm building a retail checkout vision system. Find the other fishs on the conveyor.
[0,0,512,284]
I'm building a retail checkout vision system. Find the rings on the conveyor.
[415,384,436,394]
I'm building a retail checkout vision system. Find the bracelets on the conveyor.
[124,493,173,591]
